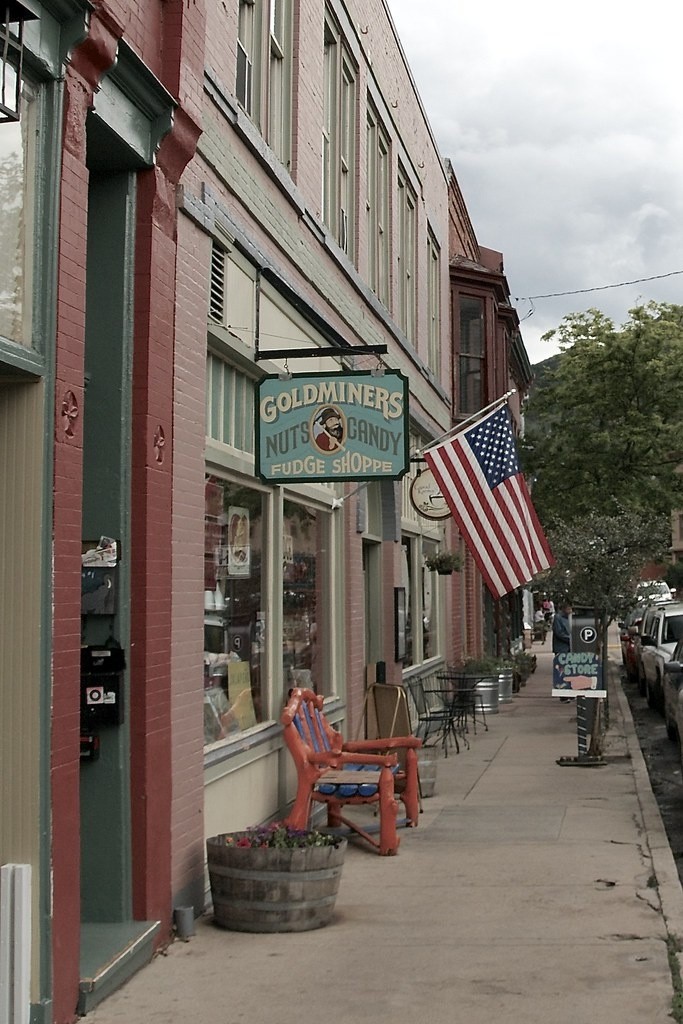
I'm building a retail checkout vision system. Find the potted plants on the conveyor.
[486,656,512,702]
[466,659,500,714]
[511,653,536,691]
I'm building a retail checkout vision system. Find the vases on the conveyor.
[205,831,347,932]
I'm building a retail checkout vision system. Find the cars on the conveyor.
[614,601,683,714]
[633,579,677,604]
[200,602,428,746]
[663,636,683,766]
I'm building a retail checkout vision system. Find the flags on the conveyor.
[424,400,555,600]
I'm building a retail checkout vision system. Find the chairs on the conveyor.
[281,688,421,856]
[407,676,459,758]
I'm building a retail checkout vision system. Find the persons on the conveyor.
[542,597,554,611]
[553,602,577,703]
[535,607,545,622]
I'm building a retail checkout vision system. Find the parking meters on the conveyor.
[554,605,610,766]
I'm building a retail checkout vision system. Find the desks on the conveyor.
[437,672,494,732]
[424,688,476,749]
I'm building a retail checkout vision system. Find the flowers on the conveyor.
[226,822,333,849]
